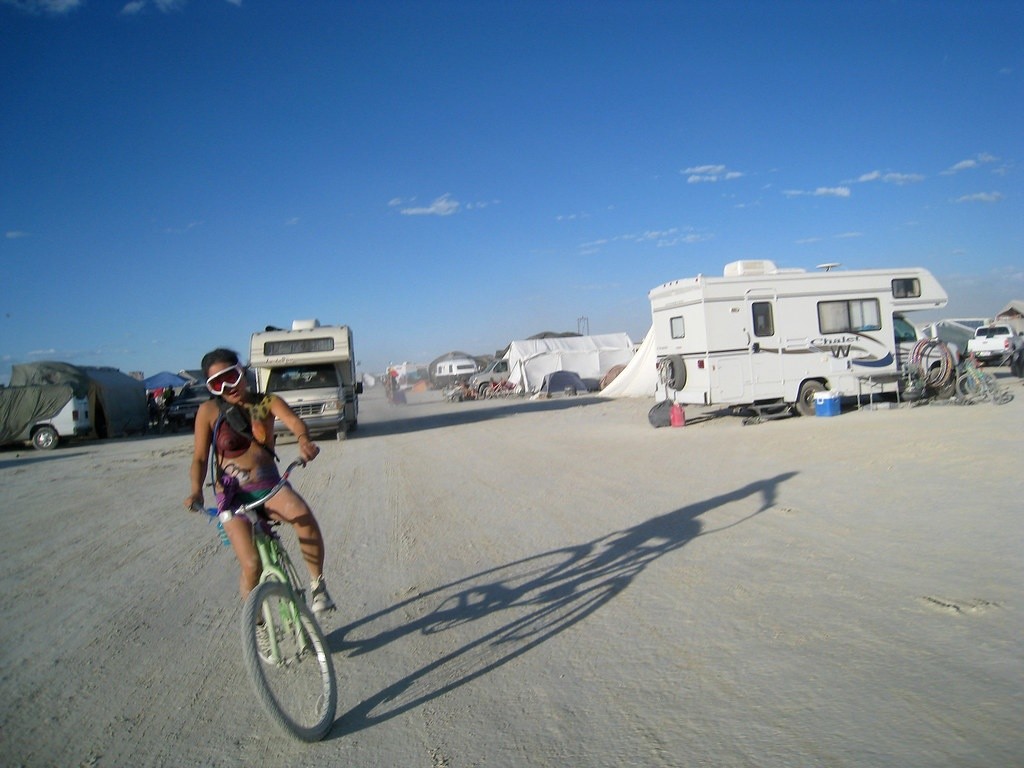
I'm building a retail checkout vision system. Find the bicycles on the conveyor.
[187,446,340,744]
[482,377,516,400]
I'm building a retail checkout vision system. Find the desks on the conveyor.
[858,371,911,410]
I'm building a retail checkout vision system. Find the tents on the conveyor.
[503,321,660,400]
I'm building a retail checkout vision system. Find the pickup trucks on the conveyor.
[966,322,1024,367]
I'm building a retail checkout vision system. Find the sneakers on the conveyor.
[254,623,282,665]
[310,574,332,611]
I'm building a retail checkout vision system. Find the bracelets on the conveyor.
[297,433,308,441]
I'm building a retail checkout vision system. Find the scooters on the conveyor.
[442,376,477,403]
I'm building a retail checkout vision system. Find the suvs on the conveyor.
[167,381,214,432]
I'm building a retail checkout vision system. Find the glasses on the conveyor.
[205,365,243,396]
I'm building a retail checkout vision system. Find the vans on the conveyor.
[434,356,481,390]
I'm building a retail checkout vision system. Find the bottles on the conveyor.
[216,519,231,546]
[670,401,685,426]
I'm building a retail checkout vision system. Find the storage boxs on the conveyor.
[814,390,842,417]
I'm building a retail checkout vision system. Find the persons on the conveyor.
[147,385,175,429]
[183,348,334,665]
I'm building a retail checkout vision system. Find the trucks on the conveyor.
[0,382,94,450]
[649,265,961,416]
[467,356,512,396]
[246,317,363,441]
